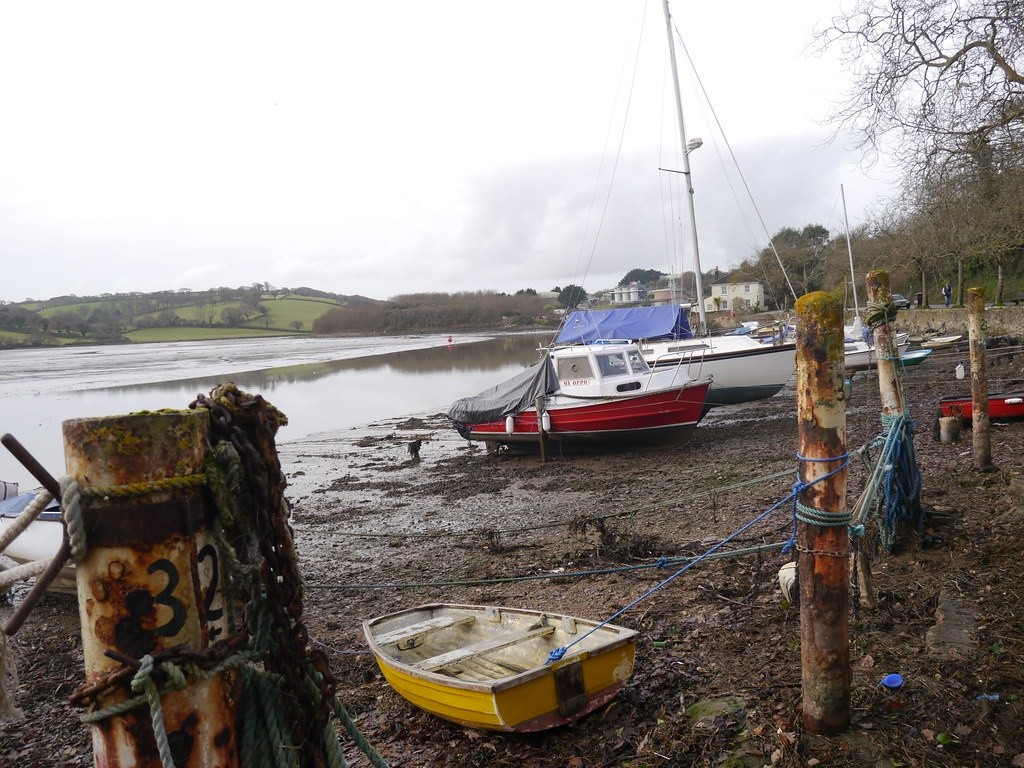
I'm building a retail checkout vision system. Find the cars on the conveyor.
[892,294,911,310]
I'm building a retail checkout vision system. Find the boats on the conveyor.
[920,334,964,351]
[1,479,78,598]
[447,1,934,456]
[939,389,1024,422]
[362,603,641,733]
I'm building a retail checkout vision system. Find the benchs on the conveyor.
[412,625,555,672]
[374,613,475,645]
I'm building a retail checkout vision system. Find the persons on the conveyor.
[942,282,953,308]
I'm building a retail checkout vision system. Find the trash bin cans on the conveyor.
[913,292,928,310]
